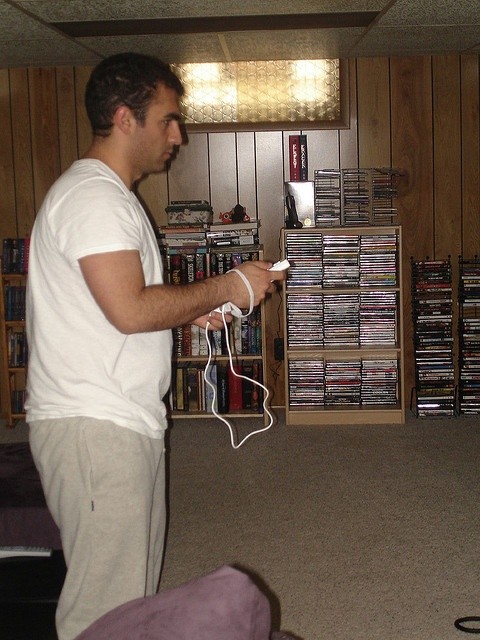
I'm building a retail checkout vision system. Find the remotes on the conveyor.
[1,541,54,562]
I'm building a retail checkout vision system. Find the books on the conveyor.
[289,134,308,182]
[4,285,26,322]
[458,258,480,416]
[164,360,263,414]
[414,260,454,418]
[2,238,29,274]
[9,372,27,414]
[154,223,209,248]
[6,327,28,368]
[172,307,262,357]
[209,219,262,248]
[161,248,258,283]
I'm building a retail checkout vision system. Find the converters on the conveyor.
[274,338,284,361]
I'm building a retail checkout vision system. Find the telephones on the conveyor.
[285,192,302,229]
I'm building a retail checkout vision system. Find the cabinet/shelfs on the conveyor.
[161,243,269,427]
[313,168,397,224]
[281,225,406,425]
[1,254,26,428]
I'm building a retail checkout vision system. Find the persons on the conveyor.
[25,51,286,639]
